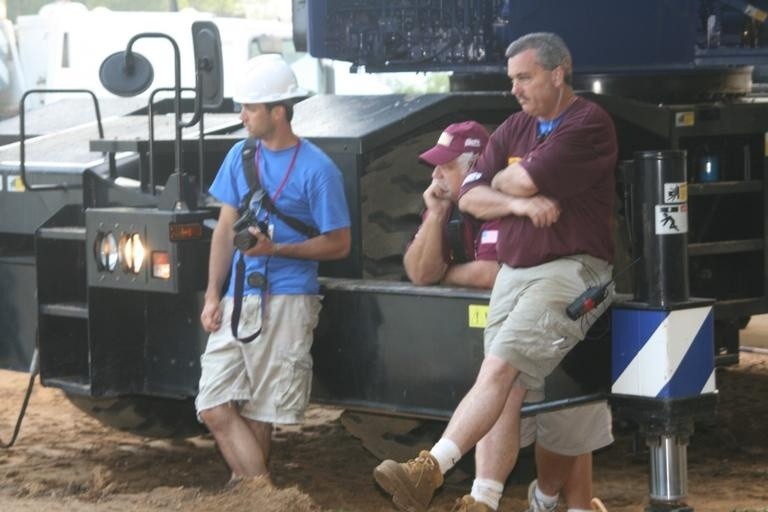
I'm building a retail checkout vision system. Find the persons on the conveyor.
[193,52,353,487]
[371,30,625,512]
[401,119,616,512]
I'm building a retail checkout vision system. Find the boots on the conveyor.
[373,450,444,512]
[453,494,496,512]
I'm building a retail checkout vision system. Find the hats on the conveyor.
[418,120,490,166]
[232,53,315,105]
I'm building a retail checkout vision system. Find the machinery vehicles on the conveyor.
[0,2,765,512]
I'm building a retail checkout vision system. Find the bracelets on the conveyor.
[274,243,281,260]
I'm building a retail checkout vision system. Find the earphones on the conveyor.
[556,71,560,81]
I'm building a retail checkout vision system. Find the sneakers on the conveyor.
[526,478,607,512]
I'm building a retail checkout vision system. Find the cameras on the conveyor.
[230,210,268,251]
